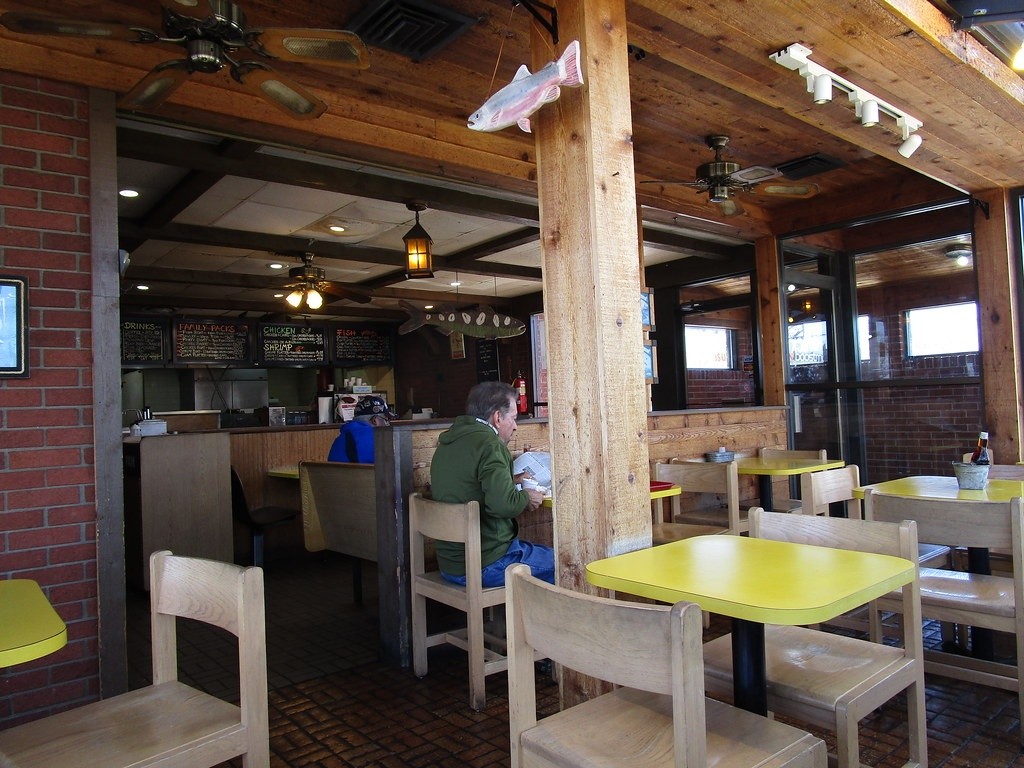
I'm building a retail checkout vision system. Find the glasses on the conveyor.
[369,415,389,424]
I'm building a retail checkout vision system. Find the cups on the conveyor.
[523,479,538,491]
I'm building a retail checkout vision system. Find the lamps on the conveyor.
[942,243,974,268]
[848,90,881,128]
[798,63,833,105]
[897,117,923,158]
[285,283,323,309]
[801,296,813,312]
[401,200,435,279]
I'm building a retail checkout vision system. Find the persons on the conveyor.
[327,396,396,465]
[430,382,556,683]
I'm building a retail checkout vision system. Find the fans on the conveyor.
[638,135,784,204]
[0,0,372,122]
[246,252,375,306]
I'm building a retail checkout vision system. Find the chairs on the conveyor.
[758,446,832,517]
[800,465,951,646]
[703,506,927,768]
[668,457,750,533]
[298,461,379,606]
[231,465,303,567]
[0,551,270,768]
[955,464,1024,563]
[863,486,1024,732]
[652,461,738,545]
[408,491,559,714]
[503,561,829,768]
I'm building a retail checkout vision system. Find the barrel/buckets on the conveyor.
[952,461,991,489]
[704,452,734,463]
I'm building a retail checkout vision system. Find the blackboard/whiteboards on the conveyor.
[257,322,329,368]
[475,339,500,384]
[172,320,254,368]
[120,316,169,369]
[330,324,394,367]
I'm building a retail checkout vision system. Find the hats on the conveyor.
[354,395,399,419]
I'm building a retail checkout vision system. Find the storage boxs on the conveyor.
[269,406,286,427]
[412,413,432,419]
[346,385,377,392]
[422,408,433,414]
[352,386,372,393]
[139,422,168,437]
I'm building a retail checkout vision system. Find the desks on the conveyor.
[721,401,754,407]
[268,469,300,479]
[850,475,1024,662]
[540,480,682,509]
[686,457,845,513]
[0,578,68,670]
[584,535,916,719]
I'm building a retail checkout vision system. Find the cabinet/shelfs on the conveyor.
[152,409,223,432]
[192,418,460,562]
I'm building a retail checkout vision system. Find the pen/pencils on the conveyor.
[136,406,151,420]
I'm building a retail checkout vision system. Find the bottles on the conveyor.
[287,412,312,425]
[971,432,990,465]
